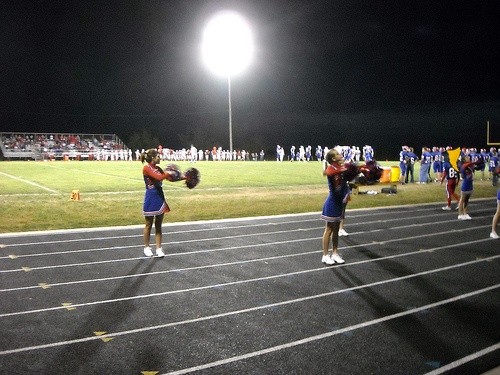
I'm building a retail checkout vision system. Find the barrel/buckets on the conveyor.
[390,165,400,181]
[381,167,392,182]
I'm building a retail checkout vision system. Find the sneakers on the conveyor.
[155,247,165,257]
[490,232,500,240]
[342,229,349,236]
[458,214,467,221]
[322,255,335,265]
[464,214,471,220]
[338,231,342,237]
[331,254,344,264]
[455,206,459,210]
[144,247,153,257]
[442,206,452,210]
